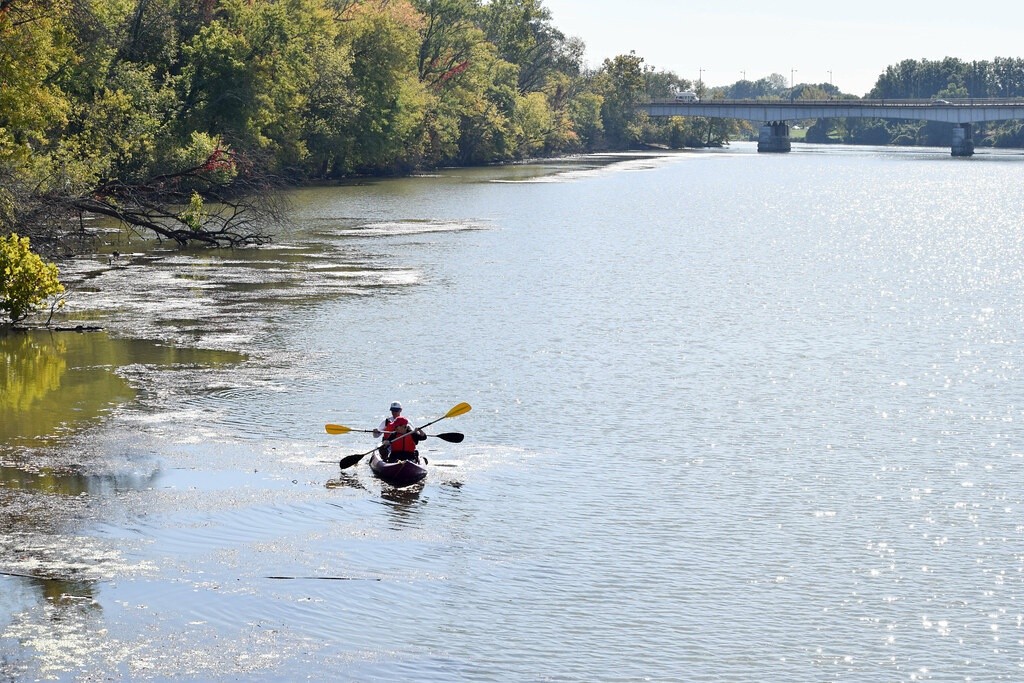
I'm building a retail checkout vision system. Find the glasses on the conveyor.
[391,408,400,412]
[394,426,398,429]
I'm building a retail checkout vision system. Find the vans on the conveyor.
[675,92,696,101]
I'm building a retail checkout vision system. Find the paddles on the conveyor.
[339,402,472,470]
[326,423,464,443]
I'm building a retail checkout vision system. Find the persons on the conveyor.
[383,416,427,463]
[373,401,414,459]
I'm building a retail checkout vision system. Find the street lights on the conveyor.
[791,70,797,103]
[740,71,745,98]
[827,70,832,100]
[700,70,705,99]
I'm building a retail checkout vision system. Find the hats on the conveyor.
[389,416,408,427]
[390,401,401,410]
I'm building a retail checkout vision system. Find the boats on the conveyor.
[370,442,427,477]
